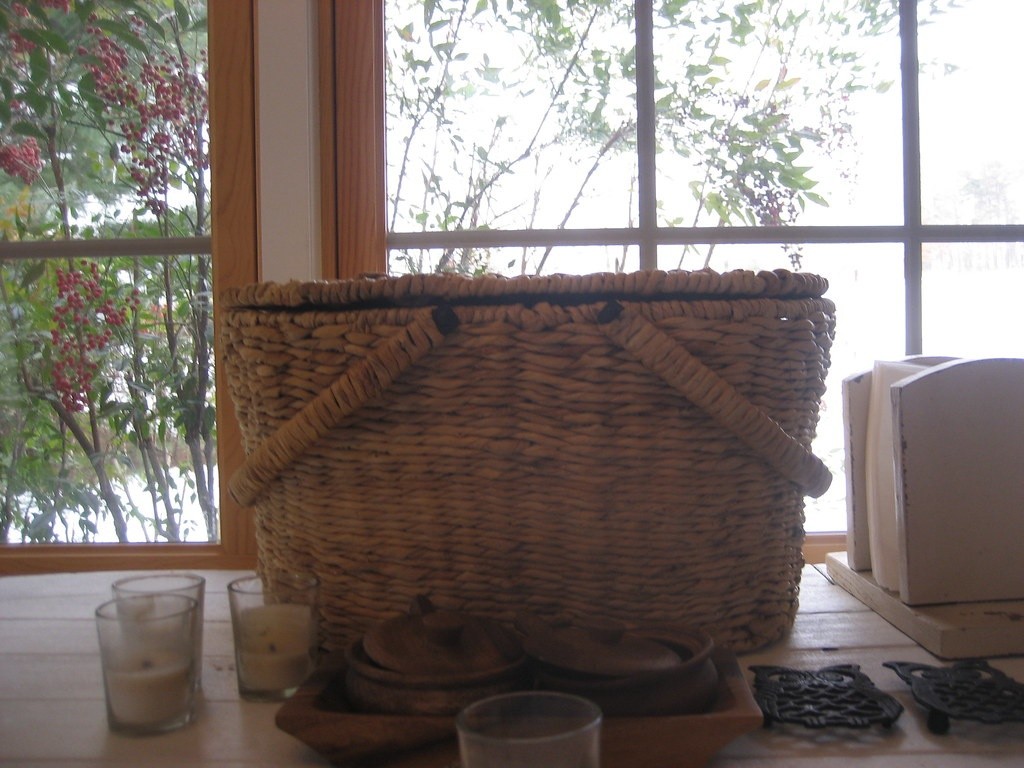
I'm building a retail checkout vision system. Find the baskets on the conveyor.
[218,268,836,653]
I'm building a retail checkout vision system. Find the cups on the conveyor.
[515,611,723,719]
[111,572,206,694]
[344,602,521,715]
[95,593,198,735]
[227,569,320,705]
[454,691,603,768]
